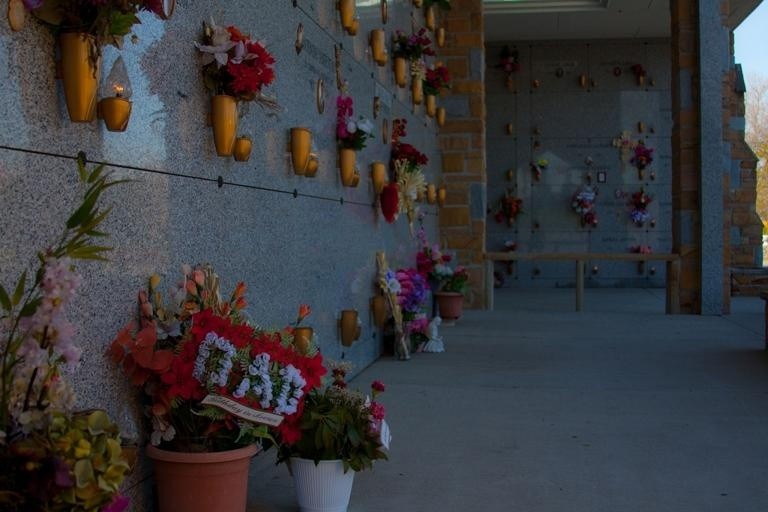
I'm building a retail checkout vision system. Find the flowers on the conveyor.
[337,96,376,151]
[102,260,328,452]
[629,139,655,169]
[391,27,436,62]
[275,356,392,476]
[194,16,282,117]
[500,240,518,252]
[425,60,453,96]
[415,210,479,304]
[487,188,528,224]
[23,0,175,78]
[410,61,426,80]
[630,245,653,255]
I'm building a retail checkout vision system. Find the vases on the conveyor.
[294,327,313,354]
[428,95,436,118]
[370,296,385,326]
[354,326,361,341]
[439,189,446,207]
[639,169,646,180]
[437,107,445,127]
[395,58,408,88]
[305,159,319,177]
[378,52,387,67]
[638,261,645,275]
[427,184,436,204]
[290,457,355,512]
[435,293,464,320]
[508,218,514,228]
[56,32,101,123]
[347,19,360,36]
[287,128,312,175]
[370,30,384,61]
[372,162,384,193]
[337,309,358,347]
[351,174,360,187]
[207,95,238,156]
[233,136,252,162]
[145,443,259,512]
[340,0,356,30]
[340,149,356,185]
[413,79,423,105]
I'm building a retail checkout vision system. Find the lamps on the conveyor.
[97,55,132,132]
